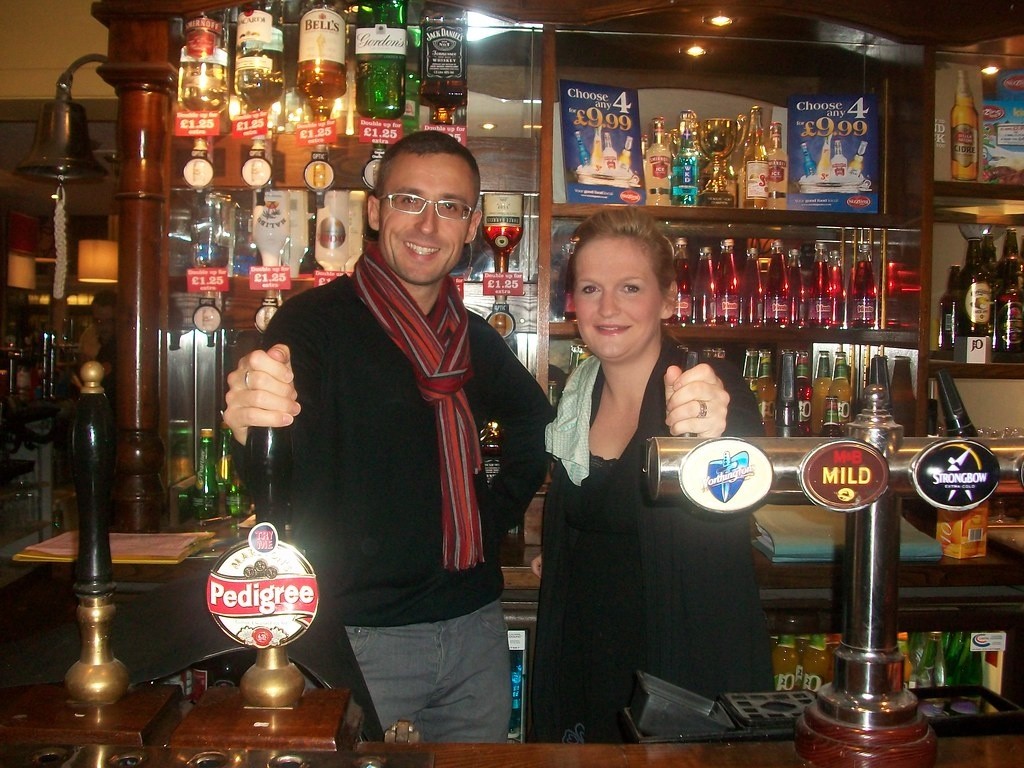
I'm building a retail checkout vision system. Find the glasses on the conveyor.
[377,192,475,222]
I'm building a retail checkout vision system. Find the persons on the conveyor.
[525,207,764,746]
[221,131,555,744]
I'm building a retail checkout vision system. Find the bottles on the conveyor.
[697,347,916,439]
[898,631,985,689]
[742,106,769,209]
[216,422,233,492]
[591,127,603,172]
[481,193,525,300]
[770,634,840,692]
[170,431,190,520]
[800,143,816,177]
[646,116,671,206]
[831,138,847,177]
[847,141,868,177]
[671,128,700,206]
[817,136,831,179]
[603,131,617,171]
[192,428,219,519]
[949,69,980,181]
[574,131,590,167]
[618,136,633,173]
[180,0,469,300]
[936,228,1024,362]
[767,121,787,211]
[666,236,878,328]
[226,456,251,517]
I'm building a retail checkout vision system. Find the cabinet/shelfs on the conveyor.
[157,6,1024,491]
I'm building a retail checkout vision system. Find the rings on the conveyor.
[697,400,707,419]
[244,370,249,391]
